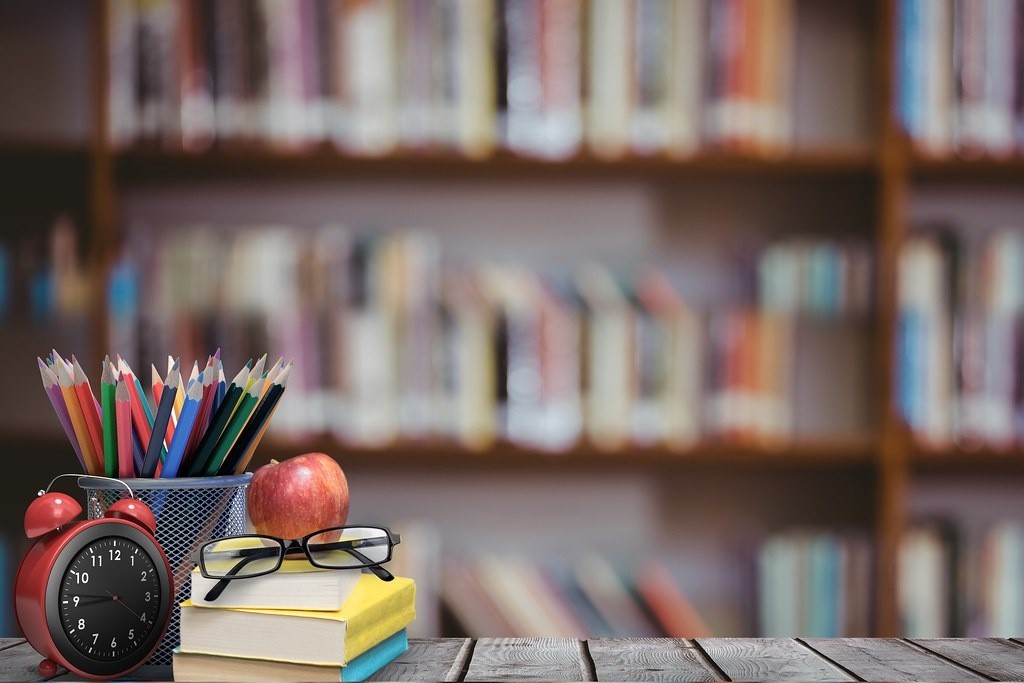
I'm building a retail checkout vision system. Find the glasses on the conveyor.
[189,526,399,601]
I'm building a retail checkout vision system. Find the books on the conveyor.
[35,0,1024,638]
[164,550,418,683]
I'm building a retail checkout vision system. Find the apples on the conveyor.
[247,452,349,560]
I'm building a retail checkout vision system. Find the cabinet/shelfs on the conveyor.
[1,1,1023,633]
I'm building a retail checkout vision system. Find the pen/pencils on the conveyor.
[37,348,293,478]
